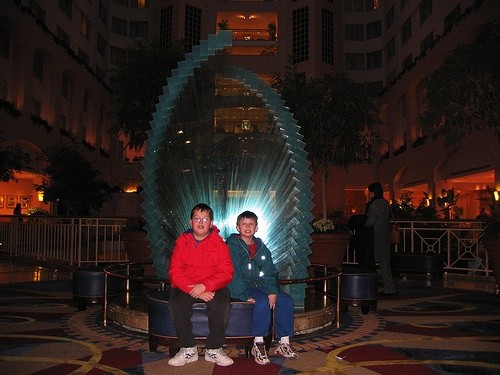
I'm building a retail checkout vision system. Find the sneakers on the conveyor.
[205,347,234,367]
[274,342,299,358]
[168,345,198,366]
[251,342,271,365]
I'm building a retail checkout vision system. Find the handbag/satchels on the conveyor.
[390,223,400,244]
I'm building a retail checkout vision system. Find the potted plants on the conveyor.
[267,55,384,274]
[413,15,500,284]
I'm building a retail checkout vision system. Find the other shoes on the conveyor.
[378,291,396,299]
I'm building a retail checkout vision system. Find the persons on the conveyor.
[167,203,234,366]
[362,182,397,296]
[13,203,22,222]
[225,211,300,365]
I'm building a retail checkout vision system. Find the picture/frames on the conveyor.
[0,195,31,209]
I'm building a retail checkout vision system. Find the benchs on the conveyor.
[148,291,273,358]
[315,266,380,314]
[71,265,144,311]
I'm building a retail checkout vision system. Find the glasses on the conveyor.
[194,218,210,222]
